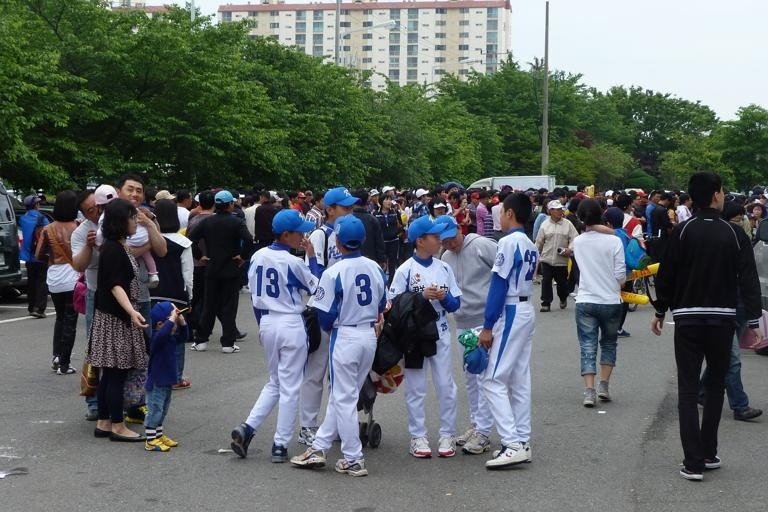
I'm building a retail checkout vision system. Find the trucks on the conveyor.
[466,175,556,194]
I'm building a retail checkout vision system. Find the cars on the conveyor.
[0,179,156,303]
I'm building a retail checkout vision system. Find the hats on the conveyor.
[25,195,42,209]
[548,199,565,211]
[466,345,488,374]
[323,187,362,209]
[637,192,649,197]
[298,190,313,198]
[408,203,458,243]
[471,194,478,200]
[272,209,315,234]
[155,190,176,202]
[215,189,244,204]
[94,184,119,206]
[334,214,366,249]
[149,302,189,325]
[416,189,429,198]
[370,185,395,197]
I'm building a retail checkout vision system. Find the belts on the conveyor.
[519,296,529,302]
[262,310,268,315]
[343,322,374,328]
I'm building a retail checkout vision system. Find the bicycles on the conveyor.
[627,229,662,313]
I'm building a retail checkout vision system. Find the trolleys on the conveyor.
[333,330,388,449]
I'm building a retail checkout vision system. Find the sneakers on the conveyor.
[148,275,160,289]
[680,456,720,480]
[85,406,178,451]
[230,423,253,458]
[51,356,77,375]
[289,447,327,466]
[271,443,288,462]
[734,407,762,420]
[298,427,316,445]
[617,329,631,338]
[484,443,532,469]
[438,434,456,458]
[26,309,47,318]
[335,458,369,477]
[457,429,490,455]
[598,380,611,402]
[410,437,433,460]
[222,332,246,353]
[540,305,550,311]
[584,389,595,406]
[560,301,566,308]
[190,341,208,352]
[172,379,190,390]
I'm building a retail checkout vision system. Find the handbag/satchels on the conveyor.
[31,218,51,253]
[79,359,97,397]
[302,309,321,352]
[73,275,87,314]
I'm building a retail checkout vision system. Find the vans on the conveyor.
[753,217,767,312]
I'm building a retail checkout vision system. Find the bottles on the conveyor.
[428,281,437,302]
[557,249,573,255]
